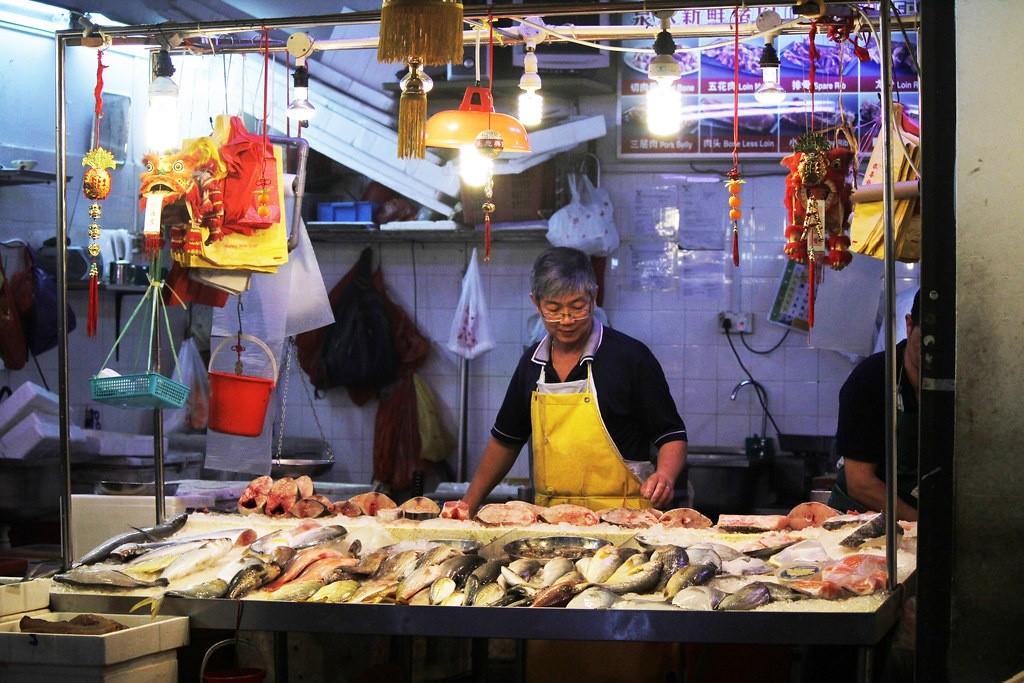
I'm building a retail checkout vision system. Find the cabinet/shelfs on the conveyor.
[306,78,614,244]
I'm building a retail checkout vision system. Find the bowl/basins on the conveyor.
[10,159,39,170]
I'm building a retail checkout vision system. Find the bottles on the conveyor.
[130,232,143,253]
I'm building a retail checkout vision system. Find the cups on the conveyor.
[110,257,136,284]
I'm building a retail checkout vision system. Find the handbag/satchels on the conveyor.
[0,245,32,371]
[23,242,77,357]
[187,337,212,428]
[446,247,497,359]
[161,338,194,434]
[294,246,460,506]
[545,174,620,257]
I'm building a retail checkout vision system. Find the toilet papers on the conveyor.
[260,173,336,338]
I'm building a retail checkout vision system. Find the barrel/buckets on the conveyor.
[207,332,278,438]
[200,639,268,683]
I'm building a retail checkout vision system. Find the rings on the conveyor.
[665,487,670,493]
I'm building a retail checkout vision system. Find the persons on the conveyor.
[830,291,920,522]
[463,247,688,513]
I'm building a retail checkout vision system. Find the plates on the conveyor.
[701,38,764,77]
[89,481,180,496]
[780,42,857,76]
[384,539,484,555]
[505,536,612,560]
[624,45,699,77]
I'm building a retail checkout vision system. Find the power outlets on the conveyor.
[718,311,754,334]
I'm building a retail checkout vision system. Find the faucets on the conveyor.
[729,379,768,439]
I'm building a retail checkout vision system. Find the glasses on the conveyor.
[537,297,591,323]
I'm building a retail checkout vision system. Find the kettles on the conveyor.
[89,228,132,279]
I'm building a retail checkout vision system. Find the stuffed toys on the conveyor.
[780,149,853,272]
[142,137,228,257]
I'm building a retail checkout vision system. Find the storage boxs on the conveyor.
[0,579,189,683]
[316,201,380,228]
[0,379,374,546]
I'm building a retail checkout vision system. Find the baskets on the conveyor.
[88,372,191,409]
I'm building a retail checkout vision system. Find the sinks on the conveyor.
[684,446,793,466]
